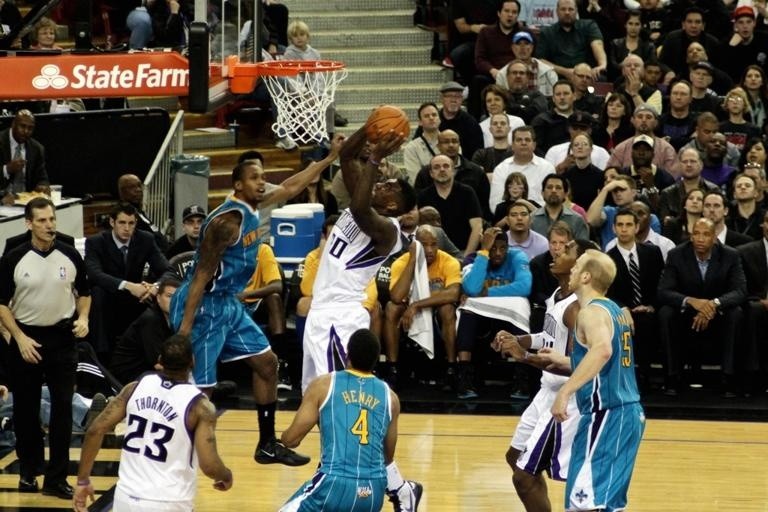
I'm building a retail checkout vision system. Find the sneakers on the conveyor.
[85,393,106,430]
[451,369,479,399]
[277,358,293,392]
[508,374,532,400]
[255,438,310,466]
[386,479,422,511]
[387,373,403,395]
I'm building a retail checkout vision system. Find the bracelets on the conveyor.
[77,478,91,484]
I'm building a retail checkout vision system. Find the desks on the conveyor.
[1,196,83,255]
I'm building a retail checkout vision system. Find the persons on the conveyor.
[277,328,402,512]
[0,197,91,500]
[70,335,233,511]
[300,125,425,512]
[539,249,645,512]
[0,2,768,436]
[489,238,603,512]
[168,130,347,465]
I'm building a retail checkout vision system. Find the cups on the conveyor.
[50,185,63,202]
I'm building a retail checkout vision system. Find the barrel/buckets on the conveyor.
[268,207,317,277]
[282,200,326,251]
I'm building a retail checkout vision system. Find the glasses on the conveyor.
[442,92,464,100]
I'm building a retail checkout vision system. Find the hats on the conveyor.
[693,61,718,76]
[438,81,465,91]
[733,5,755,21]
[566,112,595,130]
[512,31,533,45]
[631,134,656,151]
[182,203,207,223]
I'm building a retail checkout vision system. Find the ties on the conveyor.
[119,245,130,263]
[13,144,26,195]
[628,253,640,309]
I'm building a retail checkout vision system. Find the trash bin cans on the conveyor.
[171,154,210,241]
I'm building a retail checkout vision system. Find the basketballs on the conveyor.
[366,106,410,144]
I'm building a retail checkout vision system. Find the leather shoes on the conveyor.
[664,373,684,396]
[19,476,41,492]
[42,475,75,500]
[717,379,736,398]
